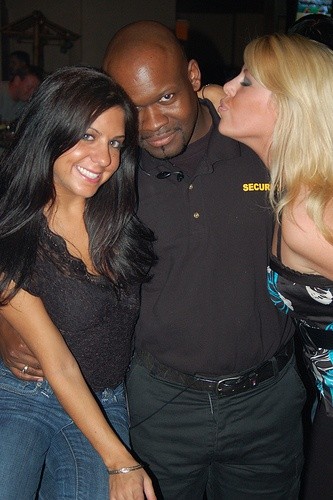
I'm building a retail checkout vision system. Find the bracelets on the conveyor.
[202,84,210,99]
[107,464,144,474]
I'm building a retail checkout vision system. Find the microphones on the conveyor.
[156,170,184,182]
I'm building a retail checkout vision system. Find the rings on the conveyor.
[19,365,29,373]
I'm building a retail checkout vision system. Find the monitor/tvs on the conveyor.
[286,0,333,27]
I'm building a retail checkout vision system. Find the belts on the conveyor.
[133,337,293,396]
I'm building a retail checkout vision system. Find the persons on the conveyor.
[0,67,157,500]
[0,50,44,122]
[196,33,333,500]
[0,21,317,500]
